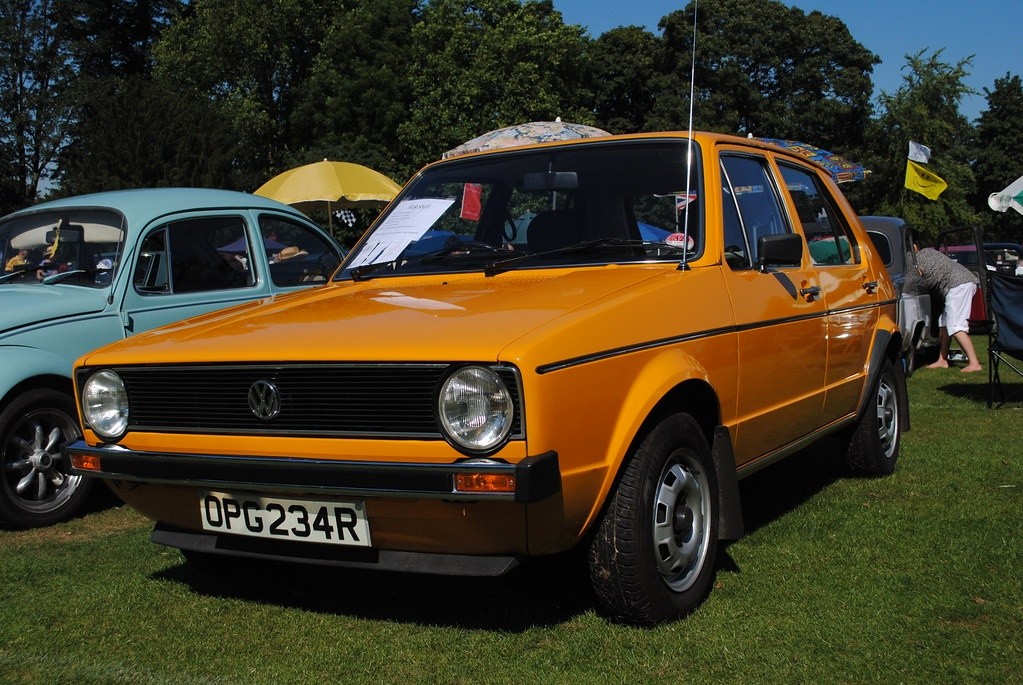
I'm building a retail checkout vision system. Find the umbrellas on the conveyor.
[442,115,612,211]
[655,131,872,197]
[253,157,404,235]
[989,176,1023,217]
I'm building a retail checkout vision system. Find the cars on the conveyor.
[395,229,474,260]
[638,214,989,377]
[0,186,352,532]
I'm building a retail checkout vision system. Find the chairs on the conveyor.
[147,249,189,292]
[986,269,1023,409]
[526,197,646,263]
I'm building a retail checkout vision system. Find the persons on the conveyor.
[912,240,983,371]
[7,248,61,283]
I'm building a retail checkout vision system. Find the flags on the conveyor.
[905,139,948,201]
[460,182,482,222]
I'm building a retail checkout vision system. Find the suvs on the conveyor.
[63,130,914,629]
